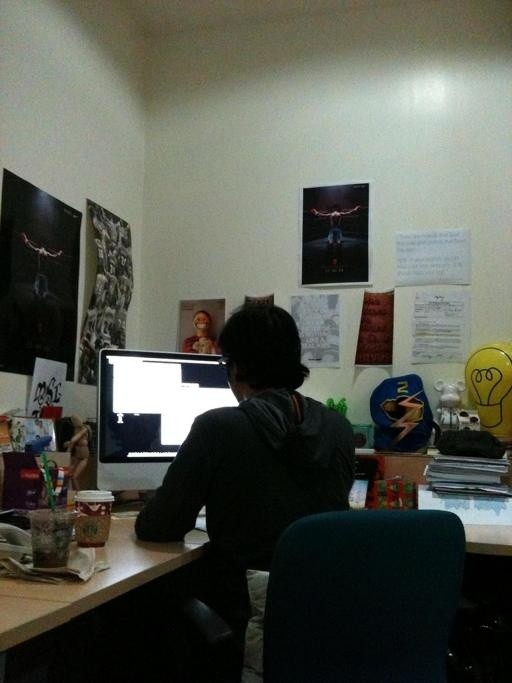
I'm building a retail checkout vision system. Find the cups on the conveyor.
[69,491,116,547]
[25,508,78,571]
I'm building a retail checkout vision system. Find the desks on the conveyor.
[0,500,512,652]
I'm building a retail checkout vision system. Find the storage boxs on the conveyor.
[380,452,432,486]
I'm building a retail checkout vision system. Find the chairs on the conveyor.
[184,507,466,682]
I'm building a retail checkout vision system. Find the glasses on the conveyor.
[219,356,227,373]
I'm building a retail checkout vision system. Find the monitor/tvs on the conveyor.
[97,348,240,490]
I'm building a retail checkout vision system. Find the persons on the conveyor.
[20,230,64,300]
[310,204,361,268]
[64,413,91,490]
[135,304,356,644]
[14,419,52,451]
[78,206,134,383]
[181,308,219,354]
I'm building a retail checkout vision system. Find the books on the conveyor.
[423,448,511,496]
